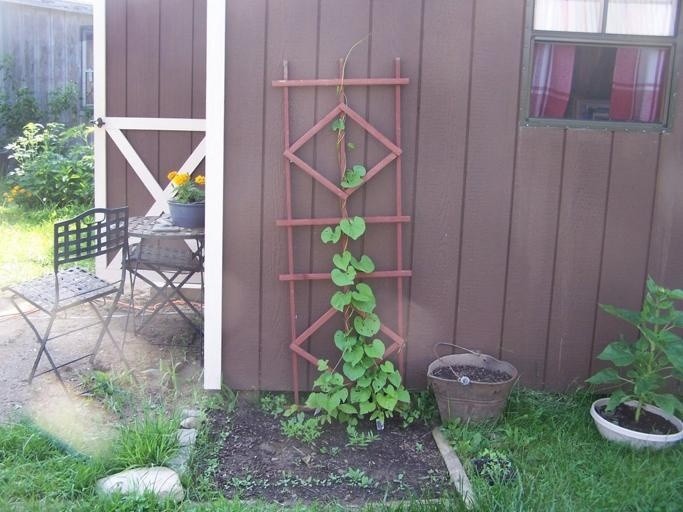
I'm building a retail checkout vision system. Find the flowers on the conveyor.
[166,170,205,200]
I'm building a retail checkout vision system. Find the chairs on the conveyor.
[0,206,139,406]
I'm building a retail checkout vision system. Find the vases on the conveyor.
[168,200,205,228]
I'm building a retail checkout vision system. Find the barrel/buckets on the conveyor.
[427,342,519,426]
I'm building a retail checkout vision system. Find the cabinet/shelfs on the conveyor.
[121,215,204,338]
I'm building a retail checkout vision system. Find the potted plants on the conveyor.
[583,275,683,451]
